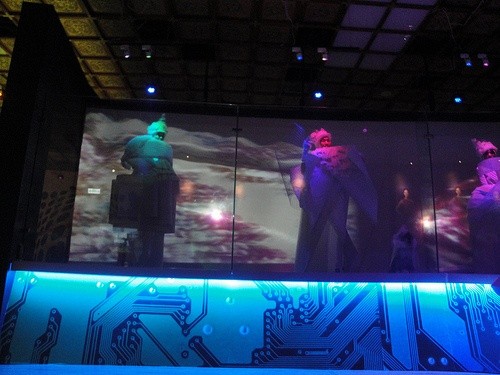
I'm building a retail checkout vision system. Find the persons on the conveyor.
[390,189,439,273]
[128,120,173,266]
[474,141,499,273]
[450,186,474,272]
[300,128,351,273]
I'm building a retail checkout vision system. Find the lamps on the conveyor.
[120,45,131,59]
[291,47,303,60]
[317,47,328,60]
[477,53,488,67]
[460,53,472,67]
[141,45,153,59]
